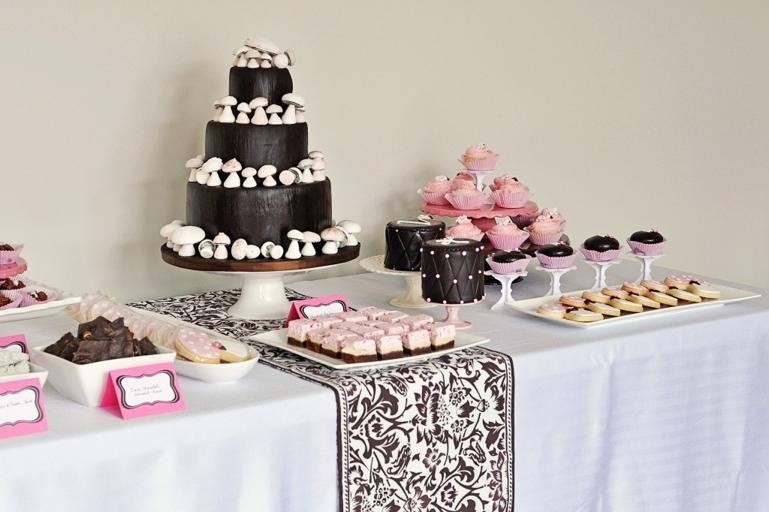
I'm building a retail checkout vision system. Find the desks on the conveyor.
[0,262,768,510]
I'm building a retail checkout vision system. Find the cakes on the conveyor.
[577,235,623,260]
[288,306,455,363]
[160,38,361,259]
[384,143,577,304]
[627,231,668,254]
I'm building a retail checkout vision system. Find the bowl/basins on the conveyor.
[1,362,48,389]
[28,337,176,408]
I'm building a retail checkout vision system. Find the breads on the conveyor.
[537,274,720,322]
[78,294,252,364]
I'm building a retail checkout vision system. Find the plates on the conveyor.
[0,280,83,320]
[130,305,260,385]
[248,303,492,374]
[507,275,763,330]
